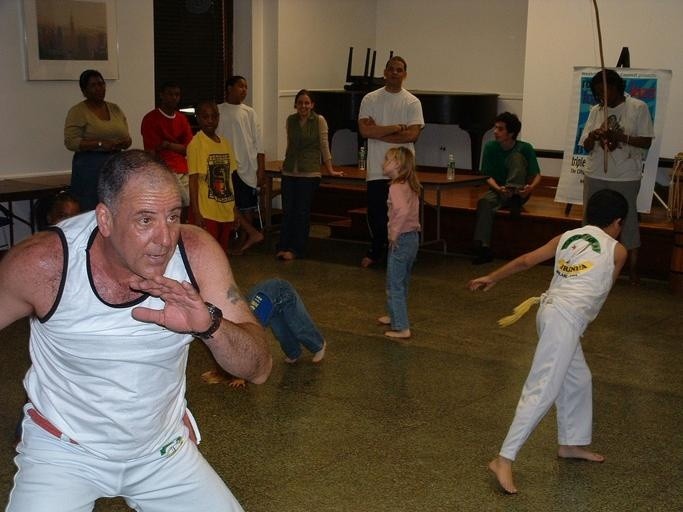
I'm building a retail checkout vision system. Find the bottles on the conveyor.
[358,147,366,170]
[447,155,455,179]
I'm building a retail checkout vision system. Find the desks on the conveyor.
[264,159,490,257]
[0,179,52,254]
[309,90,503,171]
[19,173,75,227]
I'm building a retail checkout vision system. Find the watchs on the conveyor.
[189,301,222,338]
[96,141,102,147]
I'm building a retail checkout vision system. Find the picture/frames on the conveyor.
[20,0,120,82]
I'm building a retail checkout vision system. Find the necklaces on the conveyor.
[157,107,176,119]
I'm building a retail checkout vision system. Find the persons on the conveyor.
[378,146,423,338]
[63,70,132,215]
[214,76,265,257]
[141,81,193,209]
[577,69,656,289]
[275,89,343,260]
[357,56,426,268]
[0,148,273,511]
[31,185,81,226]
[467,188,630,494]
[185,101,238,252]
[210,279,326,389]
[471,111,541,266]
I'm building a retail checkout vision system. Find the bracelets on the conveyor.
[399,125,403,132]
[404,125,407,131]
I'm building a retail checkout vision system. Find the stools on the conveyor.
[341,47,393,90]
